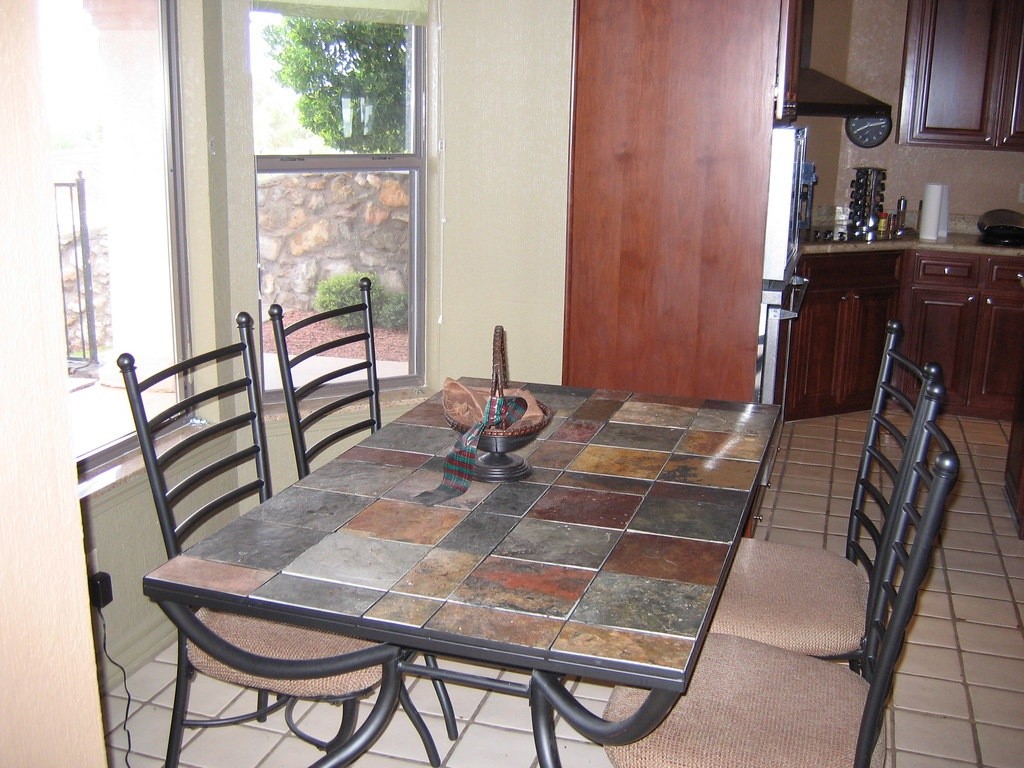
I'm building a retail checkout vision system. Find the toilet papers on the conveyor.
[918,182,949,240]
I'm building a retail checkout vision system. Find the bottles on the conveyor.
[876,212,889,241]
[888,214,897,240]
[897,196,907,229]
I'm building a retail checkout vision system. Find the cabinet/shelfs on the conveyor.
[779,248,902,424]
[896,248,1024,422]
[897,0,1024,151]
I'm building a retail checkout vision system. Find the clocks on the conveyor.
[845,117,892,149]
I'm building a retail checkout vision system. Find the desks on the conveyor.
[142,373,780,768]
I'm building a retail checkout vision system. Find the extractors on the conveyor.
[796,0,892,119]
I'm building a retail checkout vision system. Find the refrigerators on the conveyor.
[755,128,813,487]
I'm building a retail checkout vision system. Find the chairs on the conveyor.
[705,315,947,677]
[600,378,960,768]
[116,312,460,768]
[270,277,464,741]
